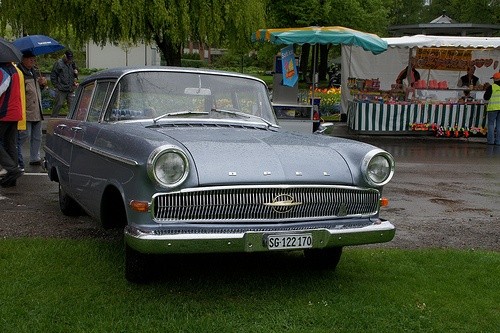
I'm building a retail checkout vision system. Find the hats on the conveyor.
[65,50,73,60]
[490,72,500,79]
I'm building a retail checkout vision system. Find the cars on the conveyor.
[44,66,396,284]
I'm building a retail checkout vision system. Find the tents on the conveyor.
[339,34,500,122]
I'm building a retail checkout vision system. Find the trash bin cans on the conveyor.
[309,98,321,119]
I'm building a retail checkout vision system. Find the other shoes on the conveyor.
[18,165,24,171]
[0,172,23,186]
[0,169,8,174]
[29,158,44,166]
[4,179,16,187]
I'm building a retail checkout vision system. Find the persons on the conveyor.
[396,66,421,85]
[0,61,23,188]
[50,49,79,118]
[457,65,481,99]
[483,72,500,145]
[17,50,47,166]
[11,62,26,173]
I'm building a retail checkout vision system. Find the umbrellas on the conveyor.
[251,26,388,120]
[10,35,64,79]
[0,39,23,62]
